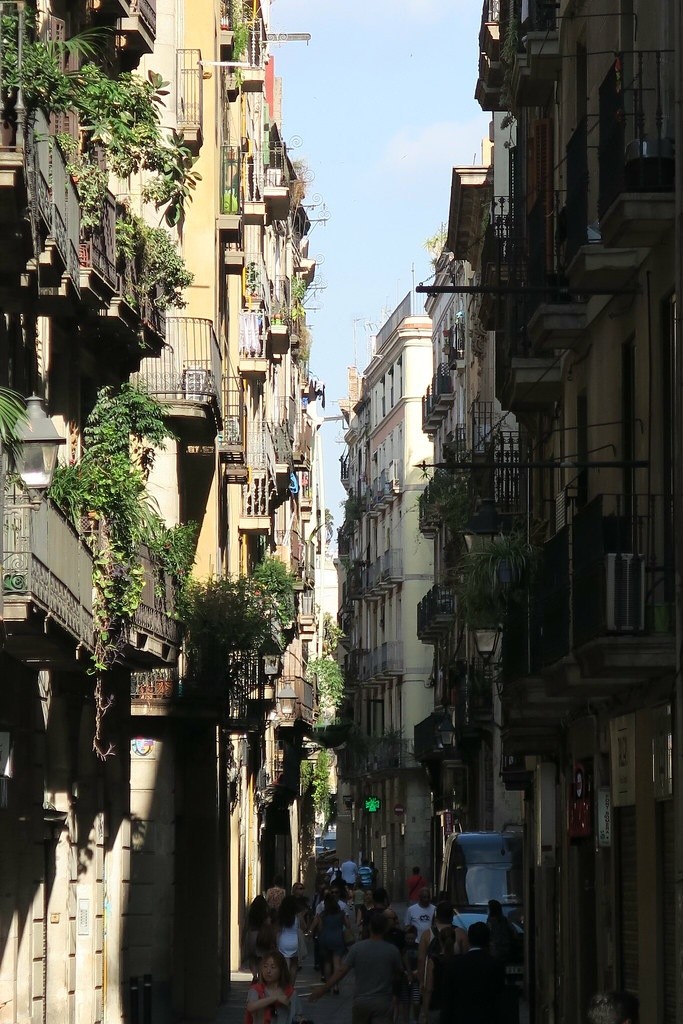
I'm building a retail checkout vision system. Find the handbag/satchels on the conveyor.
[342,927,354,946]
[256,931,276,950]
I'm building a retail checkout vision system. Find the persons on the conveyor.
[585,987,640,1024]
[246,856,517,1024]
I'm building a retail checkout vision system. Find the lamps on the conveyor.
[472,617,505,670]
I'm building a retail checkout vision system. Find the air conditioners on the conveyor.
[223,418,242,446]
[179,368,214,404]
[262,167,285,188]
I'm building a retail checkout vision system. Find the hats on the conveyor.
[330,857,338,863]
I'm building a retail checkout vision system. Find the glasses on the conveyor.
[298,887,304,890]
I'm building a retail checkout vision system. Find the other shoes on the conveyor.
[334,989,339,994]
[314,965,320,972]
[321,977,328,983]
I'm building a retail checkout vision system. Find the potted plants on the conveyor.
[441,340,451,358]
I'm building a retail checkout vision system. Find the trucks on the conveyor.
[433,830,524,948]
